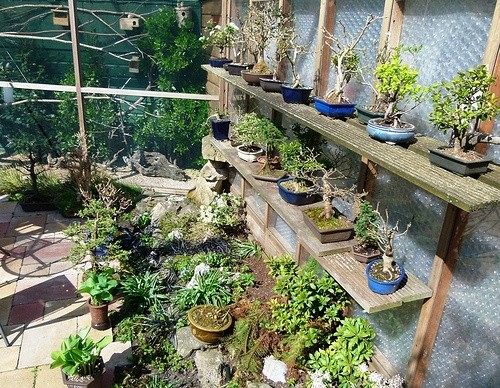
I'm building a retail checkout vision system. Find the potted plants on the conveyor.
[50,333,114,388]
[202,108,412,294]
[75,267,120,330]
[199,3,500,175]
[75,178,133,258]
[10,150,58,213]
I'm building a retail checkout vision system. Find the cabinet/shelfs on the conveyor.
[198,64,499,312]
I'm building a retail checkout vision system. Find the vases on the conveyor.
[188,303,233,344]
